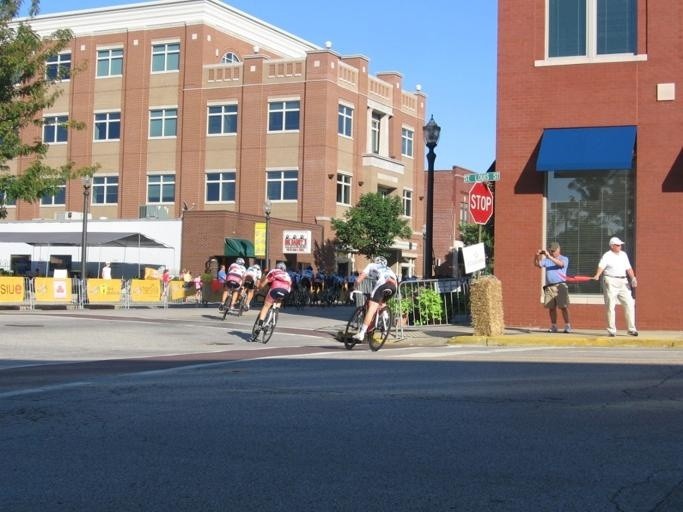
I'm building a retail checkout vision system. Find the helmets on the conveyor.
[236,258,245,265]
[375,256,387,265]
[277,263,286,270]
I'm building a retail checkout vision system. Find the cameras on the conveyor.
[540,251,545,254]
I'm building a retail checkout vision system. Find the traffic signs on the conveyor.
[463,171,500,183]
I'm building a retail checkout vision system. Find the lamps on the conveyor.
[415,83,420,91]
[251,45,260,54]
[324,40,331,49]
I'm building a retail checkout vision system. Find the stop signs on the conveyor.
[466,182,495,226]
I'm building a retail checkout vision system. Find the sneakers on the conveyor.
[628,330,638,335]
[352,333,364,341]
[548,325,572,333]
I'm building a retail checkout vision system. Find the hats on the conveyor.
[549,242,559,250]
[609,237,624,245]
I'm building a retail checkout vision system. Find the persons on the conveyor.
[535,242,572,333]
[162,268,171,297]
[351,256,397,340]
[593,236,639,336]
[181,257,359,334]
[101,261,112,280]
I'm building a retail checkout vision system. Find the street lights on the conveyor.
[264,196,273,268]
[81,171,91,303]
[422,112,443,288]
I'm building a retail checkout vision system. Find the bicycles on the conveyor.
[344,287,394,353]
[252,289,284,344]
[240,281,256,317]
[281,284,340,309]
[220,280,239,321]
